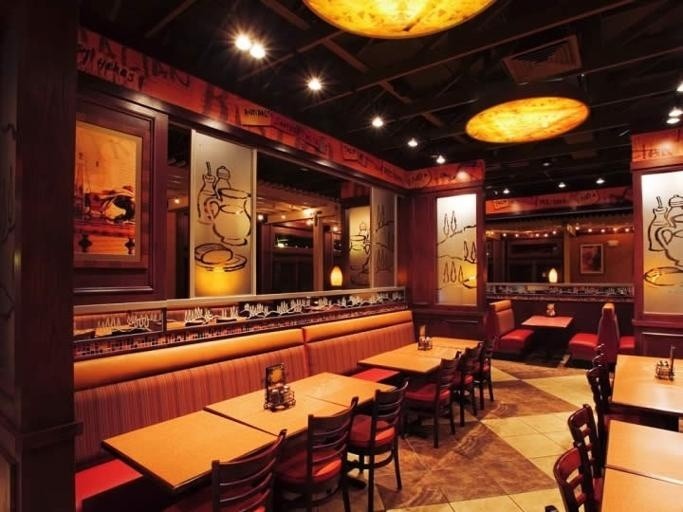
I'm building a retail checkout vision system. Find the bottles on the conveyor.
[418,336,430,348]
[281,386,293,403]
[270,385,279,403]
[655,360,668,377]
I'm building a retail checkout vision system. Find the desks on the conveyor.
[203,385,351,441]
[357,337,483,373]
[286,371,398,410]
[101,408,280,494]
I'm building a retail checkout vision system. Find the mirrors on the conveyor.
[486,208,633,281]
[254,151,372,296]
[164,119,191,300]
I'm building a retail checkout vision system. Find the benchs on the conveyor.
[486,295,636,367]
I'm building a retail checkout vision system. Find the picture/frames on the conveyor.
[76,109,151,274]
[580,242,603,274]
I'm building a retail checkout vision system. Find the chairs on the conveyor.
[280,397,359,512]
[167,429,286,511]
[535,345,683,512]
[351,381,409,511]
[469,337,494,410]
[405,351,462,449]
[453,344,477,428]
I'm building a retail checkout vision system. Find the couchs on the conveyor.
[73,328,311,512]
[303,310,415,377]
